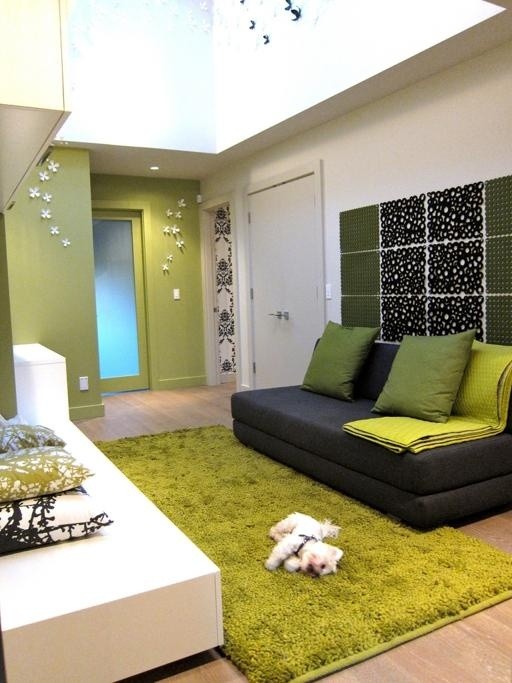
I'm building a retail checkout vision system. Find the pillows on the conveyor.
[371,329,476,424]
[301,320,382,402]
[0,423,113,555]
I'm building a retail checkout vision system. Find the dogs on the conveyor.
[262,510,345,581]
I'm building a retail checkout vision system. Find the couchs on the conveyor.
[232,339,512,528]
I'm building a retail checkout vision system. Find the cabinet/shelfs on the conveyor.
[0,343,223,683]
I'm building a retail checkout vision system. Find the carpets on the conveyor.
[93,425,512,683]
